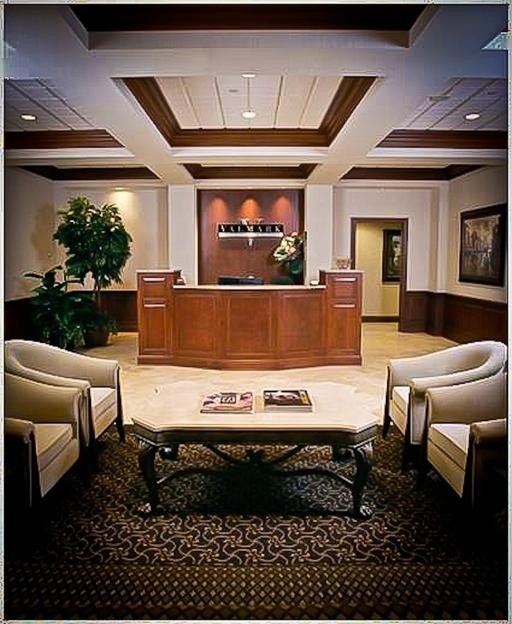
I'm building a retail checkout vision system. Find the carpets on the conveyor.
[5,424,507,621]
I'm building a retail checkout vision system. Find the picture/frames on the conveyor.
[456,200,508,288]
[380,228,404,284]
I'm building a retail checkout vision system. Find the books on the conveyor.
[263,388,312,410]
[199,389,256,413]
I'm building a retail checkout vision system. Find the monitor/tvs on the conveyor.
[217,276,264,285]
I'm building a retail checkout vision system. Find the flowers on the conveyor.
[273,228,305,264]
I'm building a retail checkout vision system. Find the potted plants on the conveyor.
[23,194,133,351]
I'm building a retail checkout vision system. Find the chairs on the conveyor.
[387,337,509,531]
[271,275,295,285]
[4,339,126,507]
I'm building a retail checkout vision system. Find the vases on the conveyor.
[285,261,302,276]
